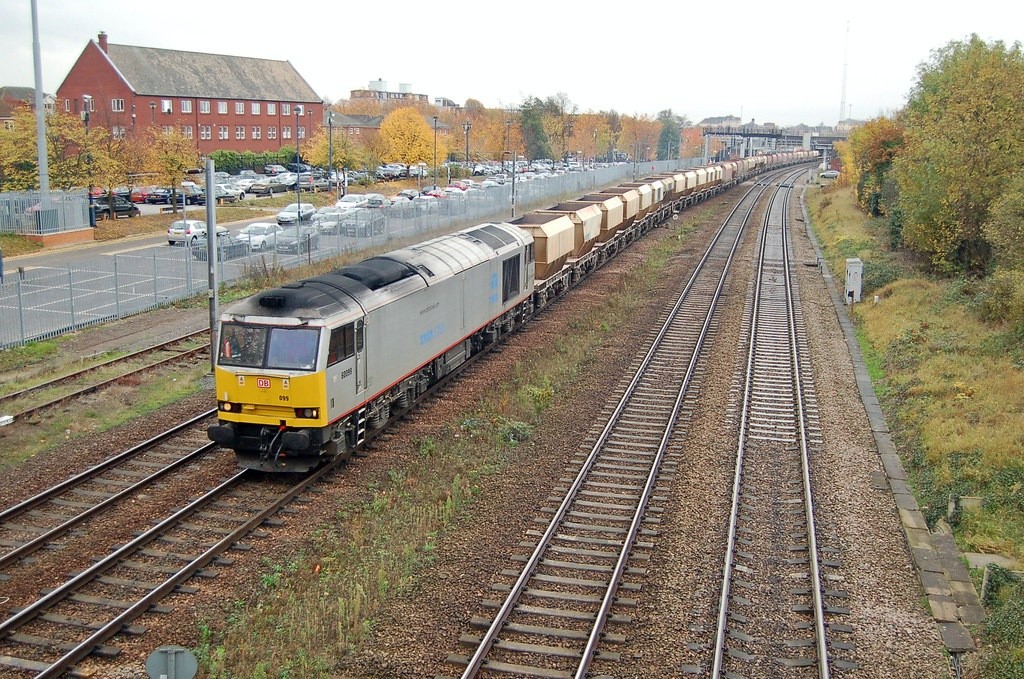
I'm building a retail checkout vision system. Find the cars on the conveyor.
[820,170,839,179]
[0,155,627,260]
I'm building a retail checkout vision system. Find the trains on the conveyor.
[207,151,819,472]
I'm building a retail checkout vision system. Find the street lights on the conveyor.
[293,106,302,226]
[462,118,472,166]
[307,109,312,143]
[506,121,511,174]
[432,116,439,190]
